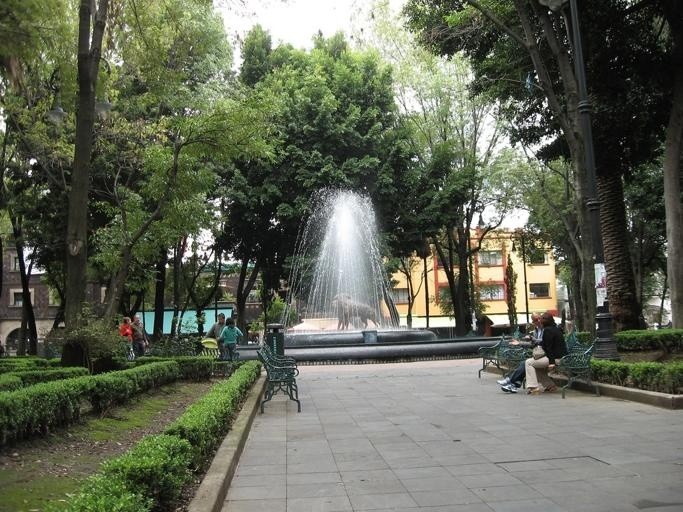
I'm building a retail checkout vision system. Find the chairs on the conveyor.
[256,341,301,413]
[479,324,601,399]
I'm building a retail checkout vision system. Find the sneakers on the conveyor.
[496,379,510,385]
[527,388,540,394]
[544,384,557,391]
[501,384,518,393]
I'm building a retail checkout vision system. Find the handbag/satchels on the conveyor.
[533,345,546,359]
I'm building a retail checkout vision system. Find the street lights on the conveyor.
[465,198,486,338]
[506,228,530,329]
[39,54,118,128]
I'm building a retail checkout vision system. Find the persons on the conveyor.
[201,312,226,362]
[213,318,244,360]
[524,311,567,397]
[117,316,135,362]
[496,311,544,393]
[130,314,148,357]
